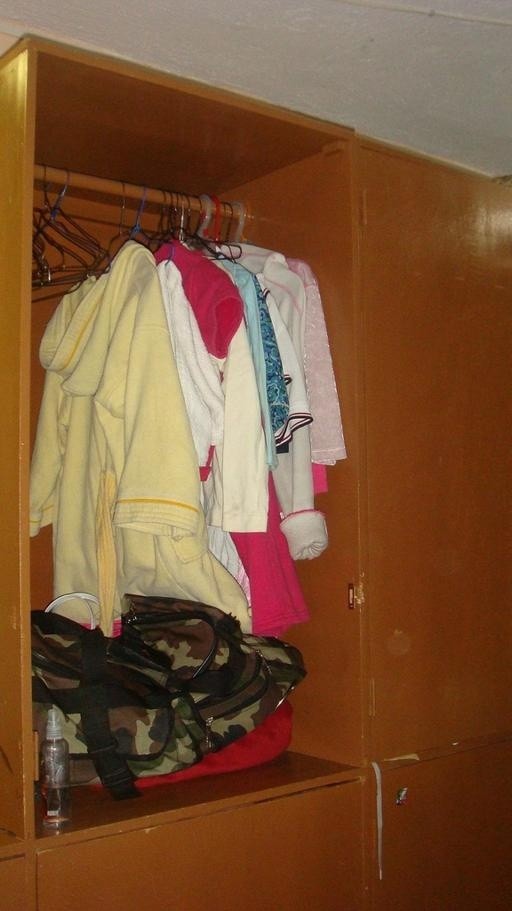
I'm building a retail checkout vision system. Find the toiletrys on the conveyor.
[42,709,70,829]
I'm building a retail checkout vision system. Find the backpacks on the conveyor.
[31,595,306,803]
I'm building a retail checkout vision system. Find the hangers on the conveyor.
[32,161,249,305]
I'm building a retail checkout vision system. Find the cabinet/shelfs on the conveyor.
[1,34,354,911]
[352,131,512,909]
